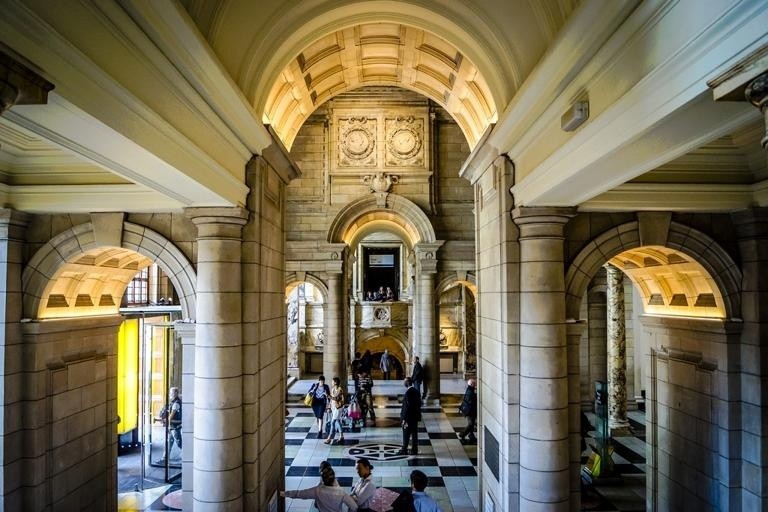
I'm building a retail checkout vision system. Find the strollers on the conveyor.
[326,390,363,433]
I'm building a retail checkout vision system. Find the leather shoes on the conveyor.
[316,432,343,444]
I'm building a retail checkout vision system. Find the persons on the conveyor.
[456,378,477,441]
[391,469,444,511]
[348,458,376,512]
[395,377,422,455]
[155,386,182,463]
[306,347,377,447]
[317,461,340,487]
[410,356,423,393]
[364,286,393,302]
[379,348,394,379]
[278,467,359,512]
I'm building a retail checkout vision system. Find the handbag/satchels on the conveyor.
[347,399,361,419]
[303,383,318,407]
[458,400,472,416]
